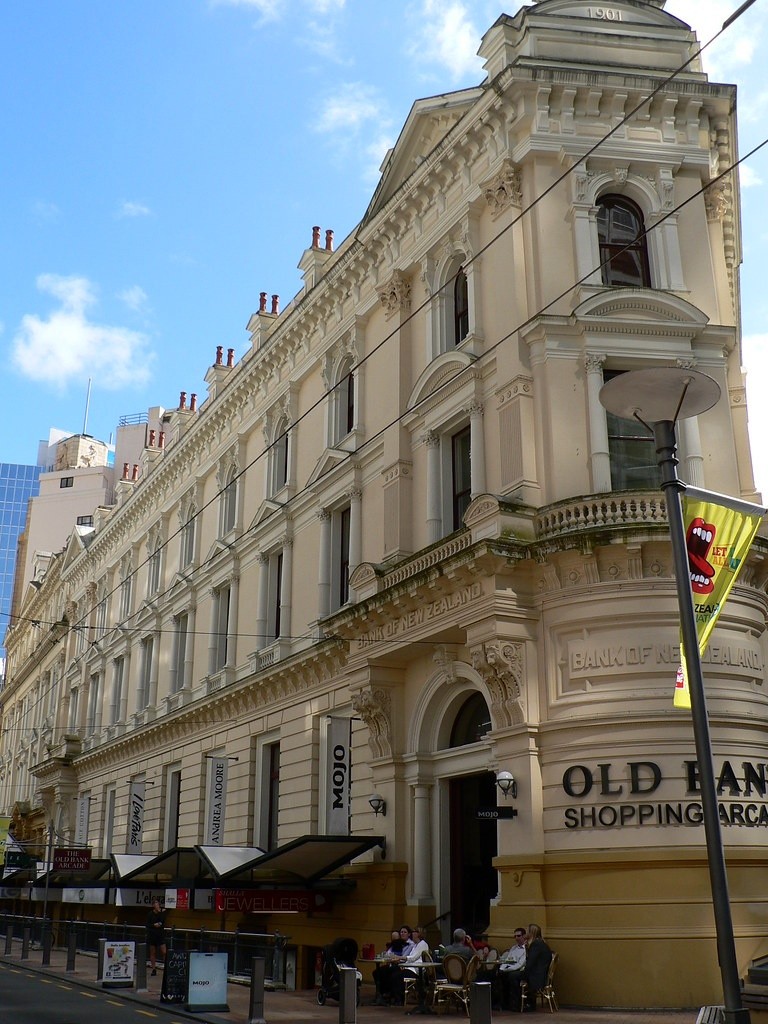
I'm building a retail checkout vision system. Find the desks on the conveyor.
[480,960,518,1010]
[359,958,400,1006]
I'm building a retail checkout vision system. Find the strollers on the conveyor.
[316,937,363,1008]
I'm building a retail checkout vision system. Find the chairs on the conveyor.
[401,949,559,1018]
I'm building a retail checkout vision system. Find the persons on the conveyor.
[372,924,551,1013]
[145,898,168,977]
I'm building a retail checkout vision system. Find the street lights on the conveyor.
[599,364,755,1024]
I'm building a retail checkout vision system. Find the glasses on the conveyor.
[514,935,523,938]
[411,930,419,933]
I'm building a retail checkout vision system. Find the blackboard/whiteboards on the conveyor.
[159,950,198,1004]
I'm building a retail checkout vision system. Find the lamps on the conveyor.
[368,793,386,819]
[496,770,517,801]
